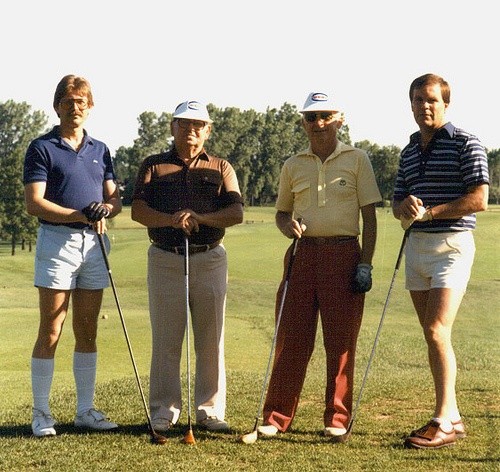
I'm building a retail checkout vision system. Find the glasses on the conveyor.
[175,118,207,130]
[59,100,89,110]
[304,112,339,123]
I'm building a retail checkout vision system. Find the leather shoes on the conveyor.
[405,420,456,449]
[411,419,467,438]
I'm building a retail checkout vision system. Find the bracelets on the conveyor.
[426,208,434,221]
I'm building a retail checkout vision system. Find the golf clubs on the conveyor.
[181,234,196,445]
[236,217,303,444]
[327,225,414,443]
[96,227,167,445]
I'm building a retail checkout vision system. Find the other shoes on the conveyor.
[258,425,277,436]
[324,427,347,436]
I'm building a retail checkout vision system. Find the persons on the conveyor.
[22,74,123,435]
[390,73,488,453]
[257,92,383,436]
[130,101,244,432]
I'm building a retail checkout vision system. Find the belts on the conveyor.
[153,240,220,254]
[301,237,357,245]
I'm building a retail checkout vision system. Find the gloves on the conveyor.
[82,201,106,222]
[350,263,374,293]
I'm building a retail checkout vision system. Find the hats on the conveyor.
[172,100,213,124]
[299,91,342,112]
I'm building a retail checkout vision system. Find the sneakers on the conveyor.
[74,408,119,431]
[200,416,227,430]
[31,414,56,436]
[148,418,172,431]
